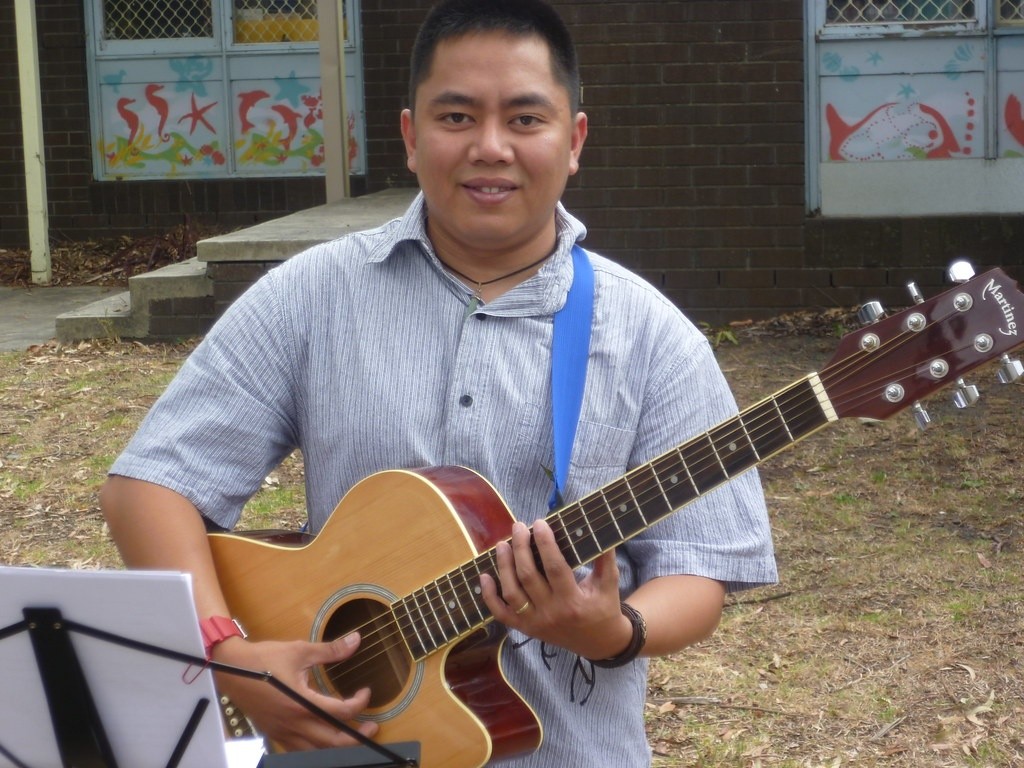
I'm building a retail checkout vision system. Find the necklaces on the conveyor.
[437,233,562,316]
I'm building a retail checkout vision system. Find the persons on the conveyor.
[97,0,779,768]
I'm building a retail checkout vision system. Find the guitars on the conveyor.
[204,256,1024,768]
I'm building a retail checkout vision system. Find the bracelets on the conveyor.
[514,600,648,705]
[198,616,248,661]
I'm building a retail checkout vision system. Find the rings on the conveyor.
[515,601,529,615]
